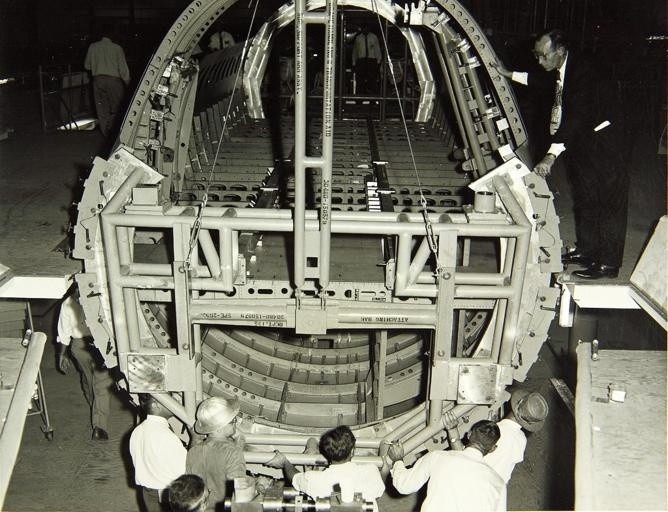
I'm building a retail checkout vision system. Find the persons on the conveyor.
[56,285,117,439]
[260,426,393,511]
[487,23,631,283]
[185,394,247,511]
[84,22,134,146]
[440,389,549,486]
[387,418,510,512]
[208,19,237,53]
[128,393,189,511]
[351,19,384,96]
[166,473,212,511]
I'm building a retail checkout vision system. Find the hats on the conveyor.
[511,389,549,432]
[195,395,240,434]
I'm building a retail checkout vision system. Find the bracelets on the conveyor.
[450,435,460,442]
[545,152,558,161]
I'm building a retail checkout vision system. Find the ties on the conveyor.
[550,70,563,135]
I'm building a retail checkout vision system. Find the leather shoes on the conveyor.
[92,427,108,441]
[561,247,595,268]
[573,262,619,279]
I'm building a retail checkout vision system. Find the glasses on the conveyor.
[228,418,237,424]
[534,48,556,60]
